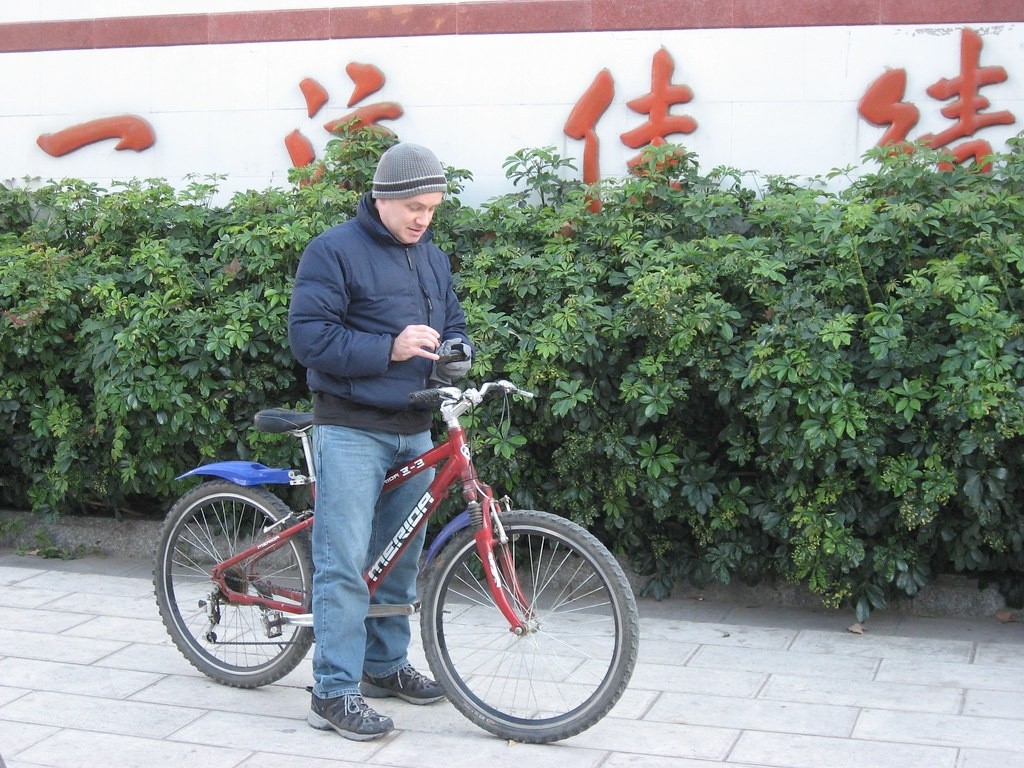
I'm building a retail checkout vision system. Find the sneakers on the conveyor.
[306,686,394,741]
[358,664,446,705]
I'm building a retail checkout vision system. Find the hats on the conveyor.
[372,143,447,199]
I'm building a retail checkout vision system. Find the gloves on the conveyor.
[429,338,471,386]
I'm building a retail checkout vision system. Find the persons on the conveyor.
[288,143,475,742]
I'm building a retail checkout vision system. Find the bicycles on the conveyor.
[152,371,642,740]
